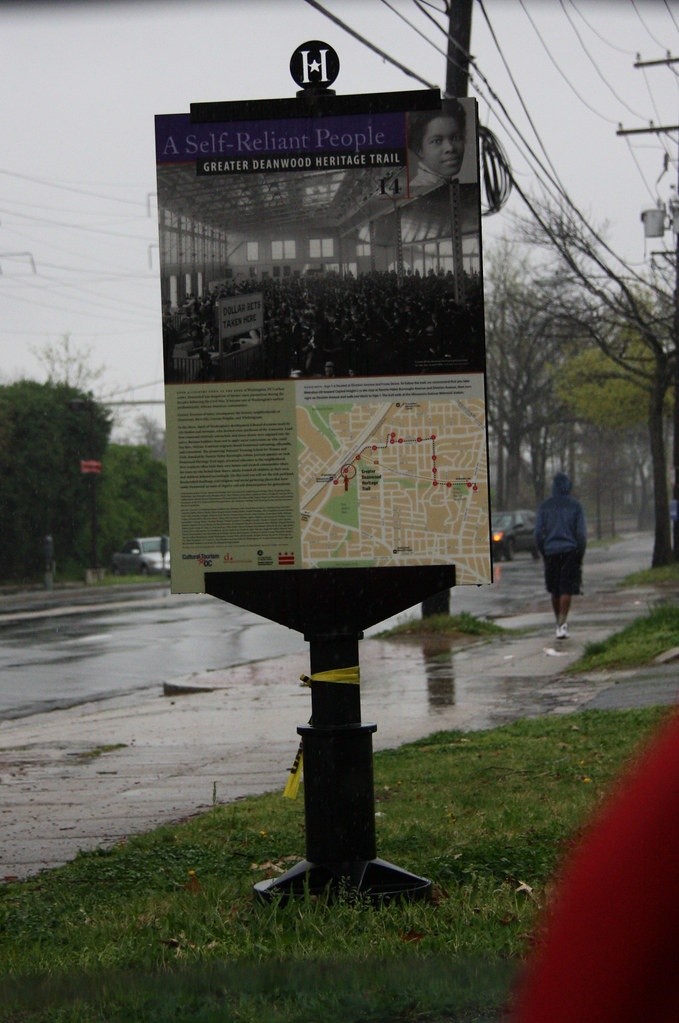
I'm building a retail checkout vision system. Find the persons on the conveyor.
[409,98,465,183]
[535,473,586,638]
[162,268,481,378]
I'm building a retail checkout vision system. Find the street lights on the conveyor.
[67,391,106,585]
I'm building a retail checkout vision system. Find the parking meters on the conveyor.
[160,537,168,597]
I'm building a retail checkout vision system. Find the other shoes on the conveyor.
[556,624,569,638]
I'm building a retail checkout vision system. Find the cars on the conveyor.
[111,536,171,577]
[490,510,540,562]
